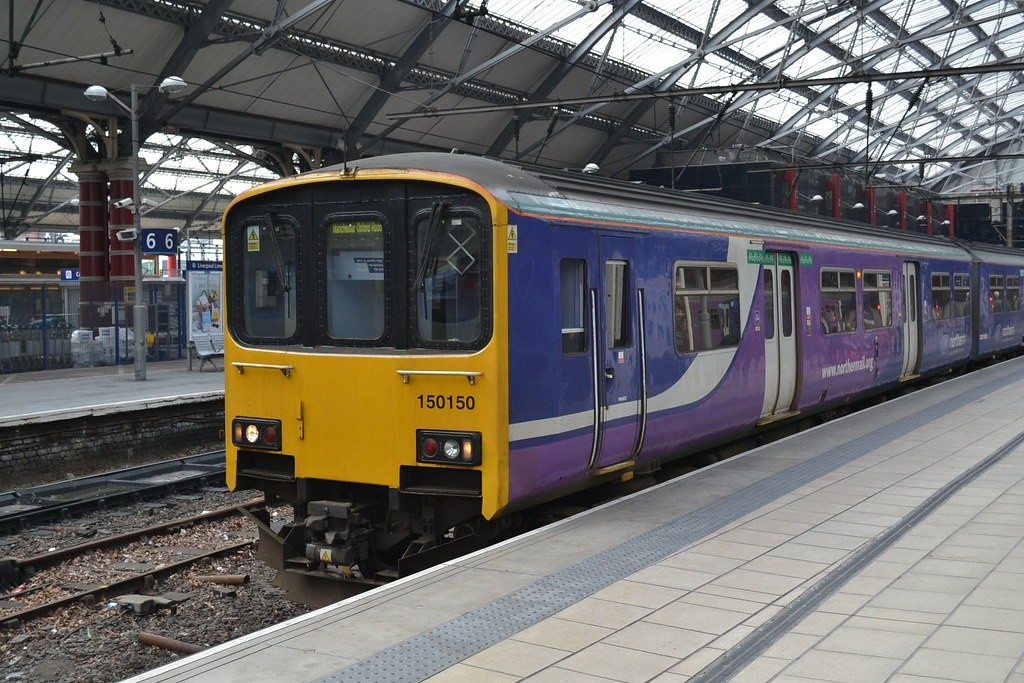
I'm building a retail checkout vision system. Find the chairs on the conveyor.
[192,332,224,372]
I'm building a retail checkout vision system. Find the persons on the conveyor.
[992,291,1003,312]
[196,289,219,330]
[1007,294,1019,311]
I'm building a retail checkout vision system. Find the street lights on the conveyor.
[899,215,926,231]
[83,75,187,381]
[868,210,898,225]
[789,194,823,211]
[925,217,950,236]
[832,202,864,219]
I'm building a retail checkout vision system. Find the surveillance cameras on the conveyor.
[114,198,133,208]
[141,198,158,207]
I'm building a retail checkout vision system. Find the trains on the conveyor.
[0,240,187,368]
[221,148,1024,609]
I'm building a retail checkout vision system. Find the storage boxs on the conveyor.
[71,327,134,367]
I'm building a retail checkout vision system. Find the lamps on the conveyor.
[931,218,950,225]
[797,192,824,203]
[876,208,898,216]
[841,201,864,209]
[906,214,926,221]
